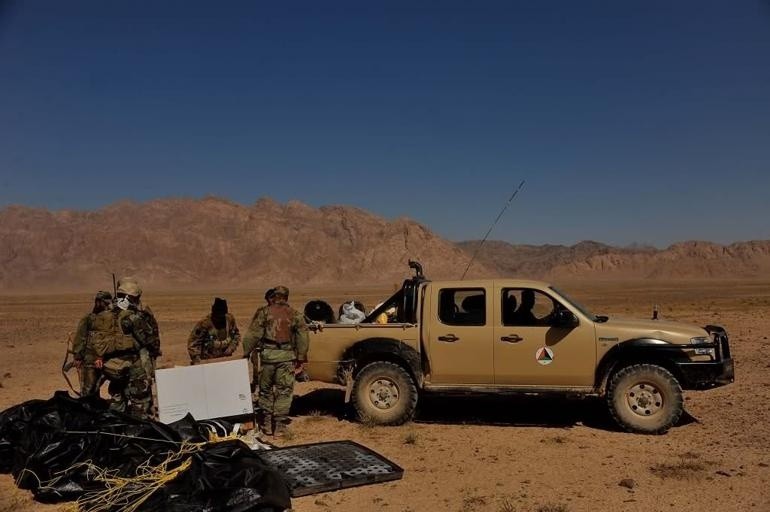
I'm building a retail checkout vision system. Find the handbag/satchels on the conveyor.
[103,358,132,383]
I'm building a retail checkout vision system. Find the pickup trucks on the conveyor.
[295,274,735,434]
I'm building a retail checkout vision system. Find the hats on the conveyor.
[211,297,228,314]
[96,291,112,303]
[265,286,289,300]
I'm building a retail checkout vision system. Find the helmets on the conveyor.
[116,277,142,297]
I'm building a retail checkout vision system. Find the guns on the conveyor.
[249,349,260,392]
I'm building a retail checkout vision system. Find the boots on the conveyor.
[262,414,274,435]
[273,422,290,440]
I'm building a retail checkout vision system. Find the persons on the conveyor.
[188,294,242,365]
[241,284,308,438]
[250,288,275,394]
[71,275,163,422]
[516,290,555,324]
